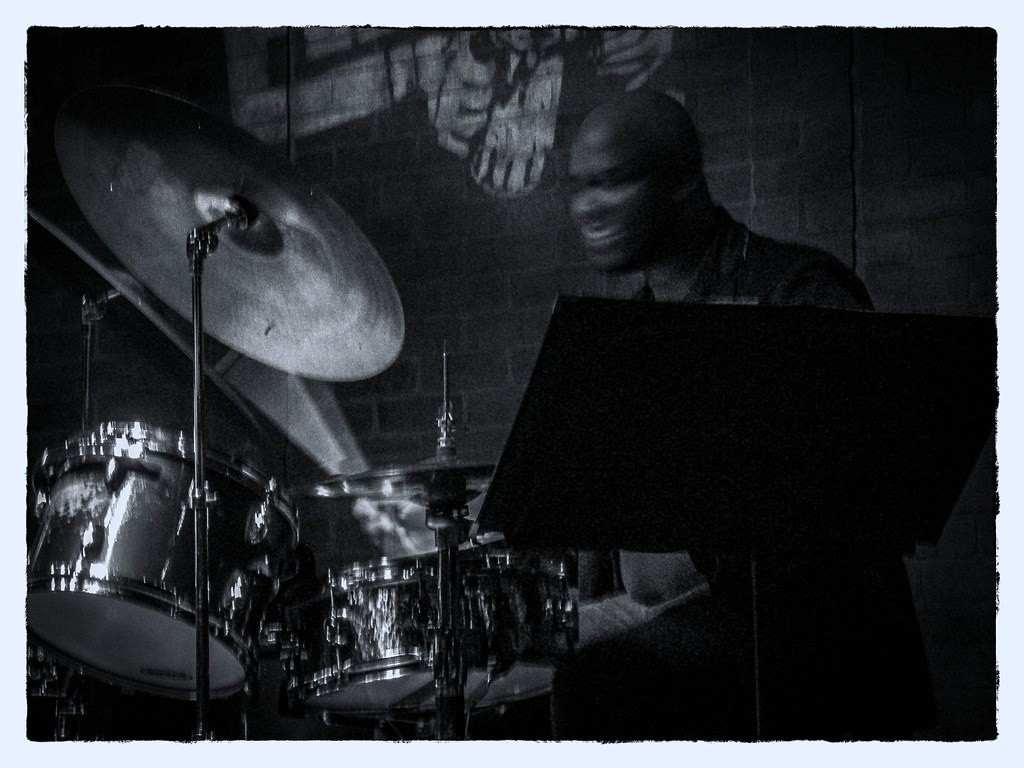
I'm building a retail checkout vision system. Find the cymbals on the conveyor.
[53,80,408,384]
[311,448,500,507]
[28,184,378,482]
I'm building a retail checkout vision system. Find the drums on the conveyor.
[32,417,311,700]
[276,544,582,726]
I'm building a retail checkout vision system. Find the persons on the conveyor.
[553,89,941,741]
